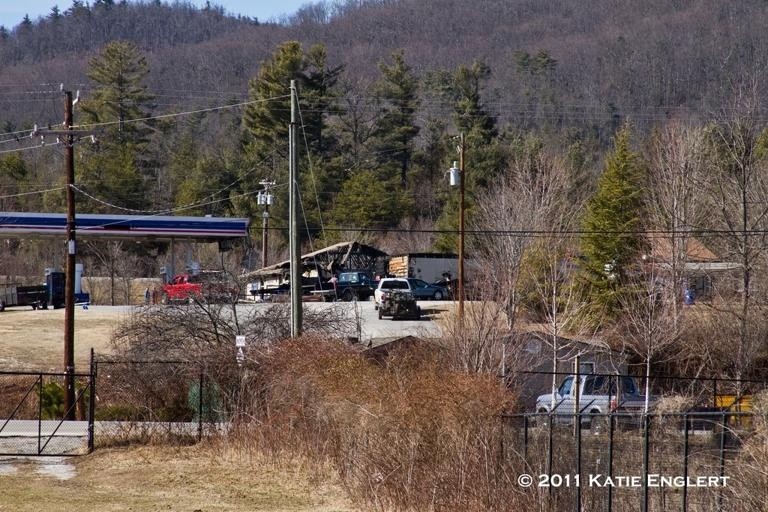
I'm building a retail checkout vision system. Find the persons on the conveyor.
[152,288,159,305]
[144,287,152,306]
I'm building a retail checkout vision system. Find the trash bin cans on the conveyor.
[684,289,695,305]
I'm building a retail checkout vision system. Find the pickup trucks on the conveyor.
[534,373,660,434]
[158,270,236,304]
[0,282,48,312]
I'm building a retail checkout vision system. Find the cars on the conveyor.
[317,270,480,321]
